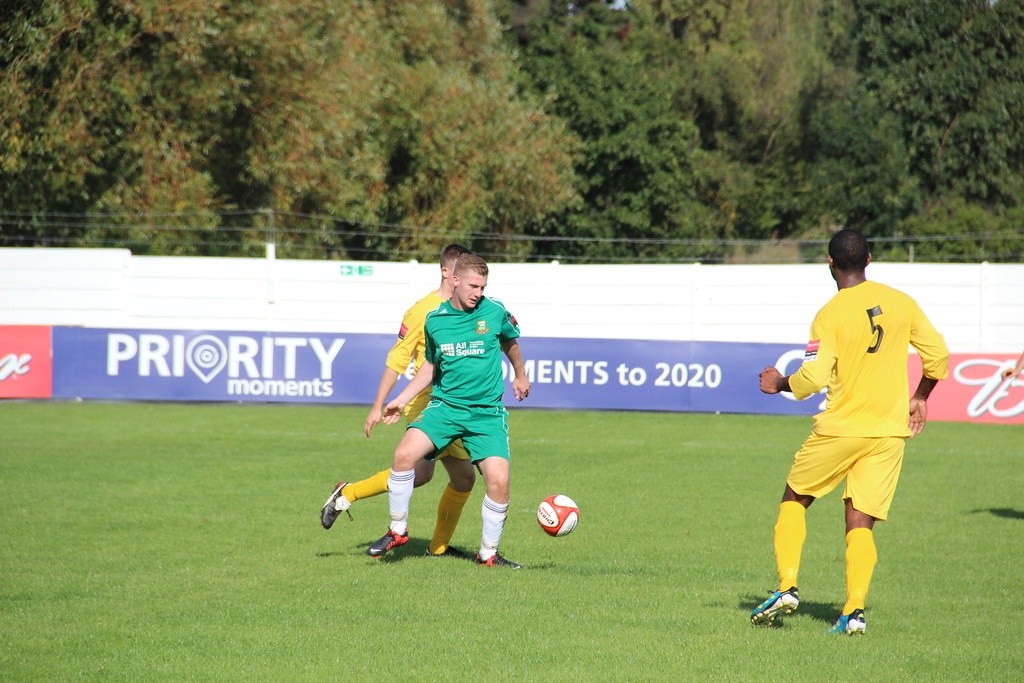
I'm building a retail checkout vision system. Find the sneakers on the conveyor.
[828,609,866,635]
[320,482,353,530]
[750,586,800,627]
[425,545,470,560]
[476,550,522,570]
[366,526,409,559]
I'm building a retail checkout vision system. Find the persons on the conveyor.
[1000,351,1024,382]
[750,229,950,638]
[320,243,475,557]
[365,255,532,569]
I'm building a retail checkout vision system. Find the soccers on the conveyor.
[535,495,580,538]
